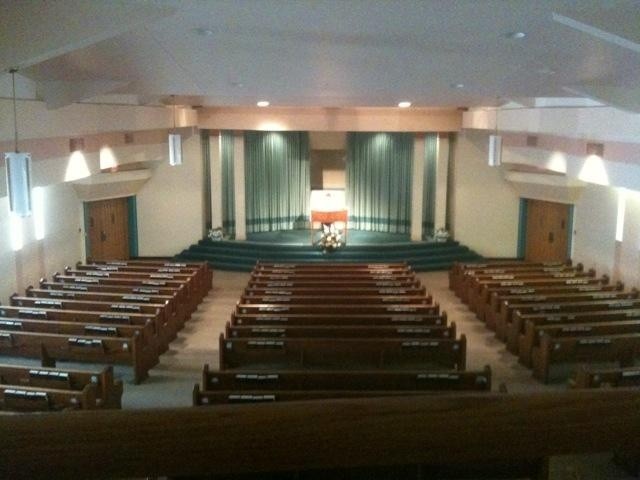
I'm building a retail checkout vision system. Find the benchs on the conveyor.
[0,254,640,480]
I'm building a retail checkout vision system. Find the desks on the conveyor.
[310,209,348,247]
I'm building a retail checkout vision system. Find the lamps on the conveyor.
[98,143,120,170]
[4,67,35,218]
[63,138,92,182]
[168,94,183,167]
[488,94,502,168]
[542,149,567,175]
[576,142,610,187]
